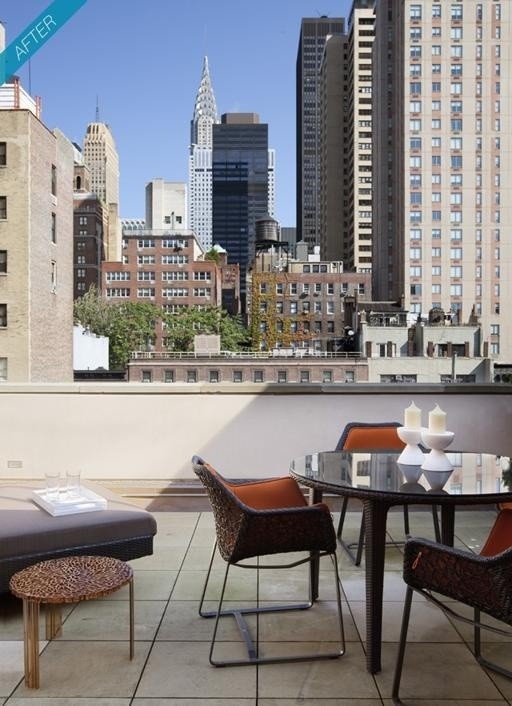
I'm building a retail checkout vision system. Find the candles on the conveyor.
[398,399,451,433]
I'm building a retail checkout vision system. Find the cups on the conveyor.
[65,467,83,498]
[43,470,61,501]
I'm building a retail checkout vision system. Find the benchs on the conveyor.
[0,483,157,588]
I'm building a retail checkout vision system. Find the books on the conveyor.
[32,483,108,517]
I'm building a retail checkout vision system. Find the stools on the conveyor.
[8,556,134,690]
